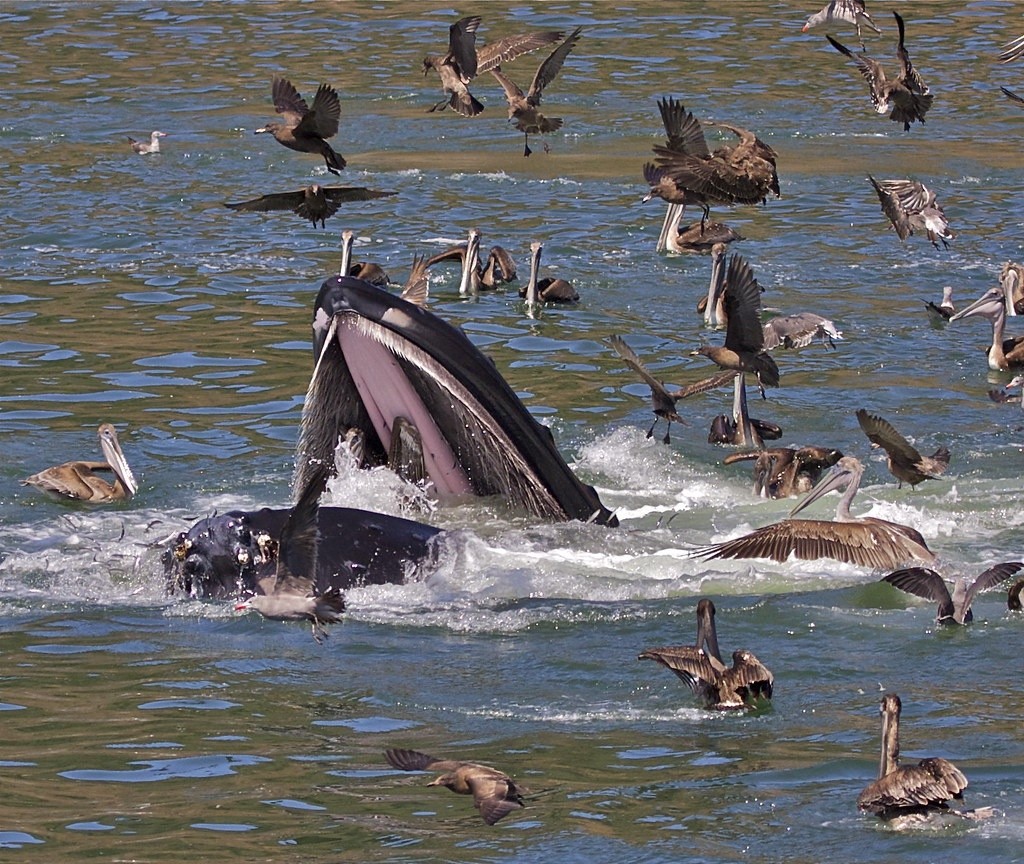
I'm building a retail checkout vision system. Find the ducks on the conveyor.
[128,131,165,156]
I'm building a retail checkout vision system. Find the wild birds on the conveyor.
[21,424,137,506]
[220,183,402,230]
[389,0,1023,828]
[232,461,347,646]
[256,74,345,178]
[338,230,391,285]
